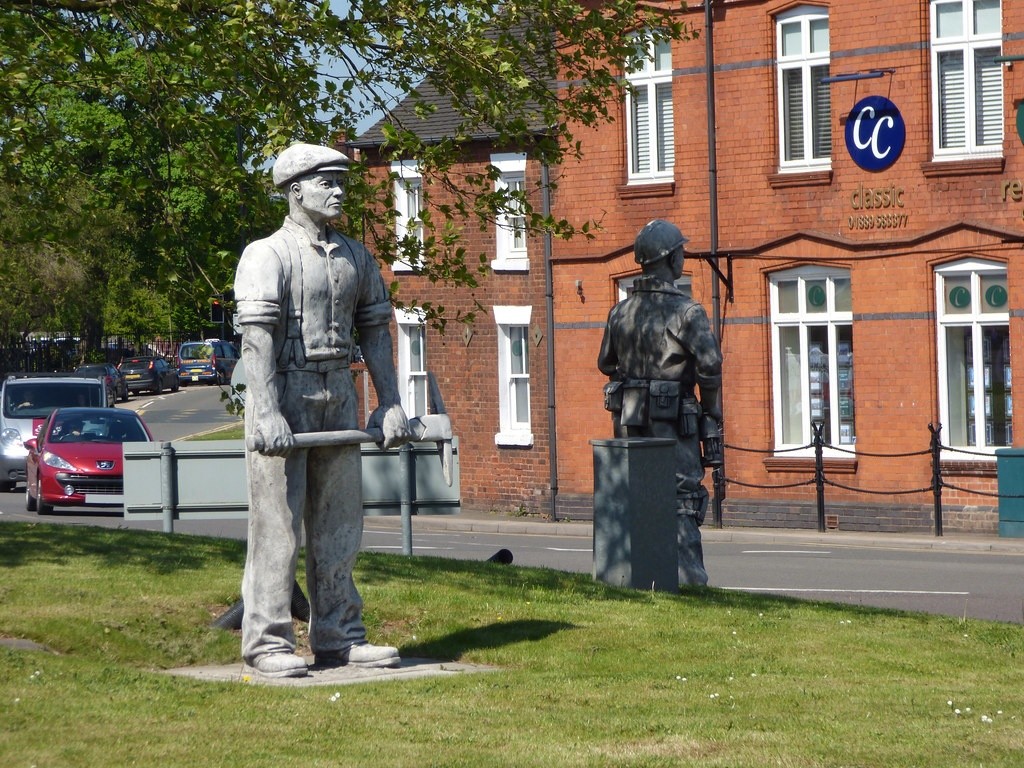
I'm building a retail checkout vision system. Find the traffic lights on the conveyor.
[211,294,225,324]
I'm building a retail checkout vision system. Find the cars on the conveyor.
[21,405,166,515]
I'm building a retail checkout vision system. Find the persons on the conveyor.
[596,219,724,590]
[10,388,35,411]
[232,142,412,678]
[59,422,82,441]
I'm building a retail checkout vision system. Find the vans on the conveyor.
[177,338,241,386]
[0,367,108,491]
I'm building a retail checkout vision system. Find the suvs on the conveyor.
[74,362,131,408]
[116,353,181,395]
